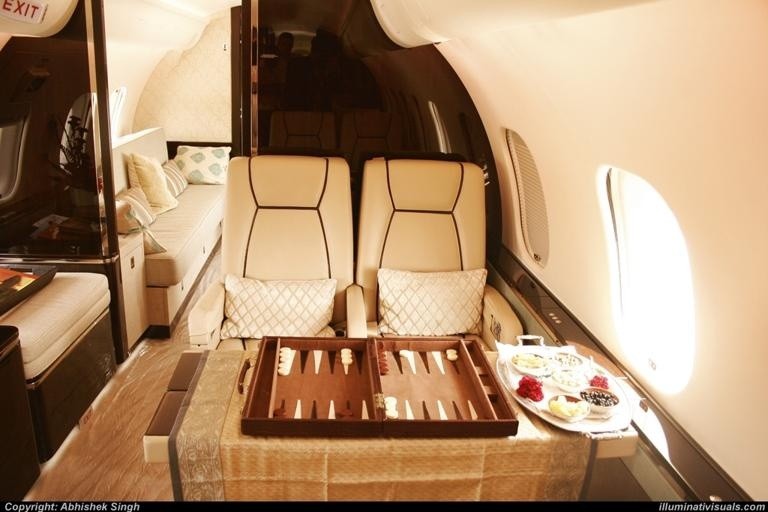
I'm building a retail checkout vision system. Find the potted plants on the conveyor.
[49,101,96,208]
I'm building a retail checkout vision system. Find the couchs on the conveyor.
[188,156,356,351]
[113,127,232,340]
[0,271,117,511]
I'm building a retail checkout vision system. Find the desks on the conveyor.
[142,350,638,499]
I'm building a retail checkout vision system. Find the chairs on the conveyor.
[355,159,524,351]
[268,109,338,152]
[340,109,403,157]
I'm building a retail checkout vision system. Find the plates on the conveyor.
[553,351,586,373]
[508,348,552,376]
[498,344,634,433]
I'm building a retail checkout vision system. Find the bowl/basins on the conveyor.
[548,394,589,422]
[579,387,619,416]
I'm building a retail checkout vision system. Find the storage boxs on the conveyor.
[230,332,520,439]
[115,232,152,364]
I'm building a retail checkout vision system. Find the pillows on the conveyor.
[162,159,189,200]
[116,186,157,232]
[116,201,168,255]
[376,268,488,337]
[124,152,179,216]
[172,145,232,185]
[219,273,337,339]
